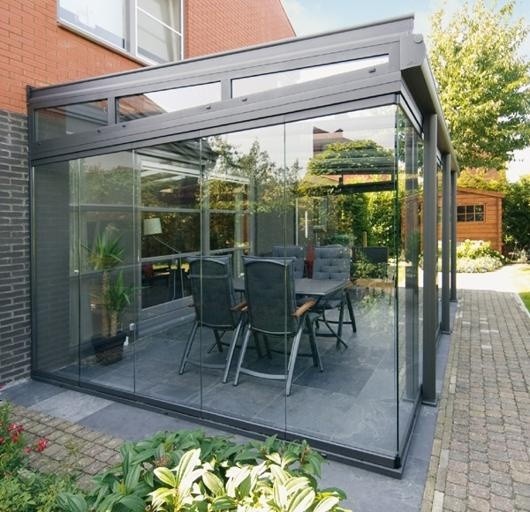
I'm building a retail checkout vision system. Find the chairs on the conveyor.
[177,241,358,397]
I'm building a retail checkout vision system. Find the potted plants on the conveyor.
[78,230,152,367]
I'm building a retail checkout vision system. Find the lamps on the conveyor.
[141,216,181,252]
